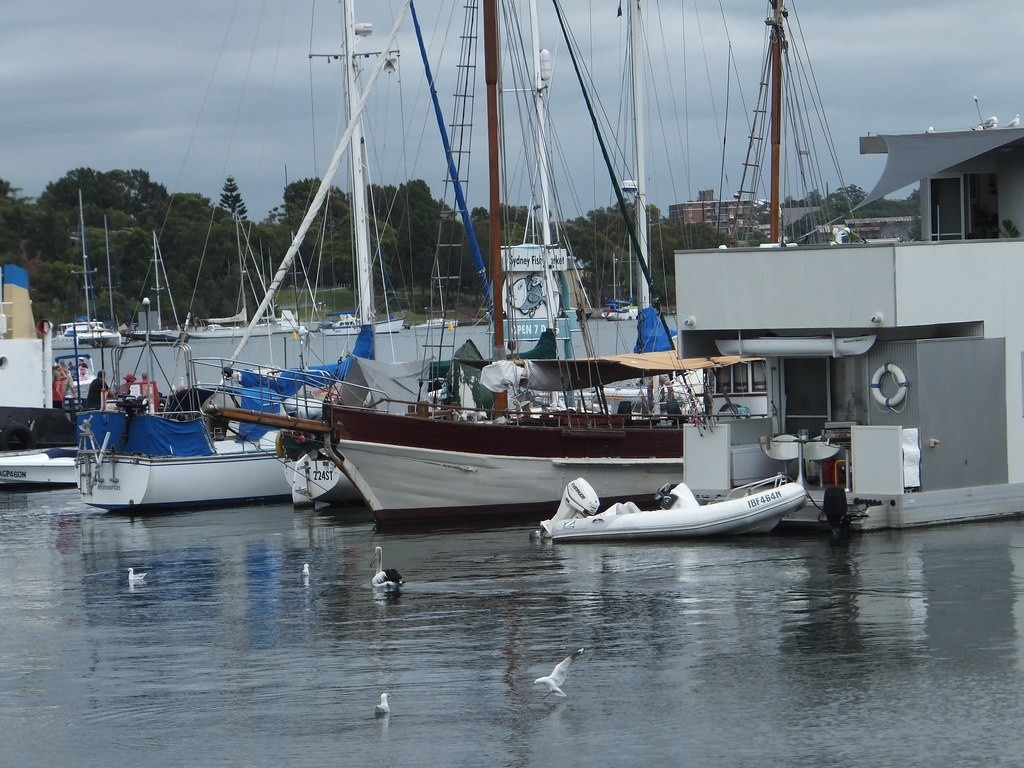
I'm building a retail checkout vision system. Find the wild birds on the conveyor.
[374,692,391,713]
[532,647,587,700]
[1004,113,1020,129]
[301,563,310,576]
[369,545,405,589]
[128,567,147,579]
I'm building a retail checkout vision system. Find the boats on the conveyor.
[55,353,98,403]
[539,478,807,539]
[0,448,79,484]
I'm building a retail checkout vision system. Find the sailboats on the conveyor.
[317,264,405,336]
[409,259,458,330]
[73,0,676,515]
[601,233,638,321]
[187,0,867,530]
[52,188,121,350]
[121,230,188,341]
[189,209,277,338]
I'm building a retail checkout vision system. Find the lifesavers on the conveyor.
[2,422,36,451]
[871,363,907,407]
[648,375,674,410]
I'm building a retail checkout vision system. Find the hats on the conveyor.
[78,357,84,359]
[122,374,137,382]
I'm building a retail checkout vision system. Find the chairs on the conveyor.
[764,435,799,484]
[802,436,841,491]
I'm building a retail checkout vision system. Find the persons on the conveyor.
[79,359,88,377]
[120,374,137,392]
[87,371,115,409]
[68,362,77,381]
[52,366,76,408]
